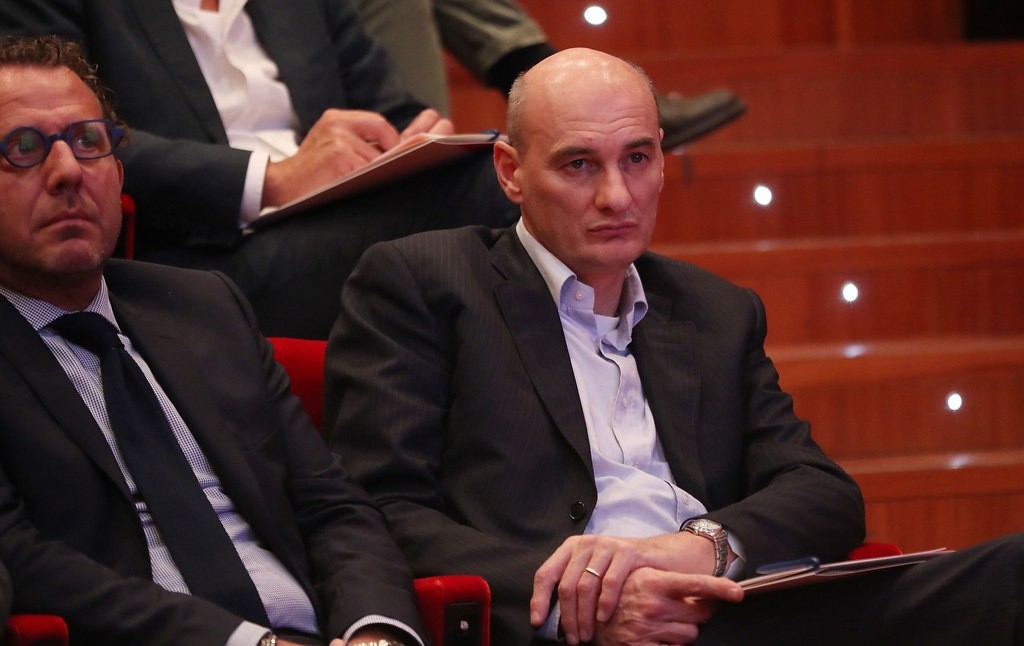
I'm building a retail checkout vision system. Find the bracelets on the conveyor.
[350,640,405,646]
[260,633,277,646]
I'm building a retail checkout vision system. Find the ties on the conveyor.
[44,310,271,630]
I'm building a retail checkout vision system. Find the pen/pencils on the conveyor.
[480,127,502,142]
[755,557,821,575]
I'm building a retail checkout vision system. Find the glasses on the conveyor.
[0,119,124,168]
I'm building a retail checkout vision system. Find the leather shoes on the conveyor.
[655,87,745,151]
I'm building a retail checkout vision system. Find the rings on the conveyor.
[585,568,604,580]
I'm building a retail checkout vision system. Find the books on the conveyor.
[735,547,957,592]
[244,129,509,232]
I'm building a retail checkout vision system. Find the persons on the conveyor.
[0,34,430,646]
[324,47,1024,646]
[0,0,745,342]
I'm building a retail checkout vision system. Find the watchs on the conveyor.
[681,519,728,577]
